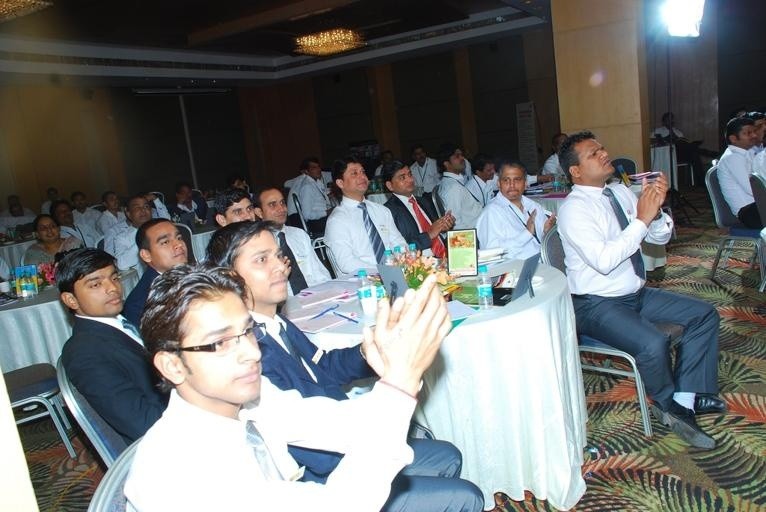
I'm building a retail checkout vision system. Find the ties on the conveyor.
[356,202,387,265]
[121,317,146,342]
[278,232,308,296]
[602,188,645,281]
[408,197,445,258]
[279,323,315,383]
[245,420,285,483]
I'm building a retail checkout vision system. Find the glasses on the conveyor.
[158,323,268,356]
[39,222,57,231]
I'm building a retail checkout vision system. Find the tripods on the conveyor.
[665,37,701,225]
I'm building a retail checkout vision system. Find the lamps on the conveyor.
[129,86,232,97]
[293,13,368,57]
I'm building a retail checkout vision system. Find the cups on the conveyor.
[357,286,377,315]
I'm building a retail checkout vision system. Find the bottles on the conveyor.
[369,178,385,193]
[382,249,395,265]
[553,174,560,193]
[408,243,419,258]
[477,263,493,310]
[19,273,28,297]
[8,269,19,298]
[561,174,567,192]
[565,179,571,193]
[24,272,37,299]
[6,227,17,239]
[394,245,403,253]
[355,269,378,317]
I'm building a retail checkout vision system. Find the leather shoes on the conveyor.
[650,392,717,450]
[663,393,728,413]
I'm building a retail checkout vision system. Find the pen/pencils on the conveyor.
[332,312,358,324]
[311,304,339,318]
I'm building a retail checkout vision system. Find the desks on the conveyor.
[651,144,678,192]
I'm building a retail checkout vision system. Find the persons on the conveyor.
[649,112,685,138]
[203,221,485,512]
[556,131,726,451]
[202,179,259,235]
[380,160,455,254]
[284,158,335,253]
[52,246,168,451]
[0,188,101,287]
[120,218,196,336]
[717,118,765,229]
[477,163,557,274]
[123,264,452,510]
[254,187,332,299]
[96,183,208,275]
[745,112,766,169]
[374,134,570,235]
[323,158,410,283]
